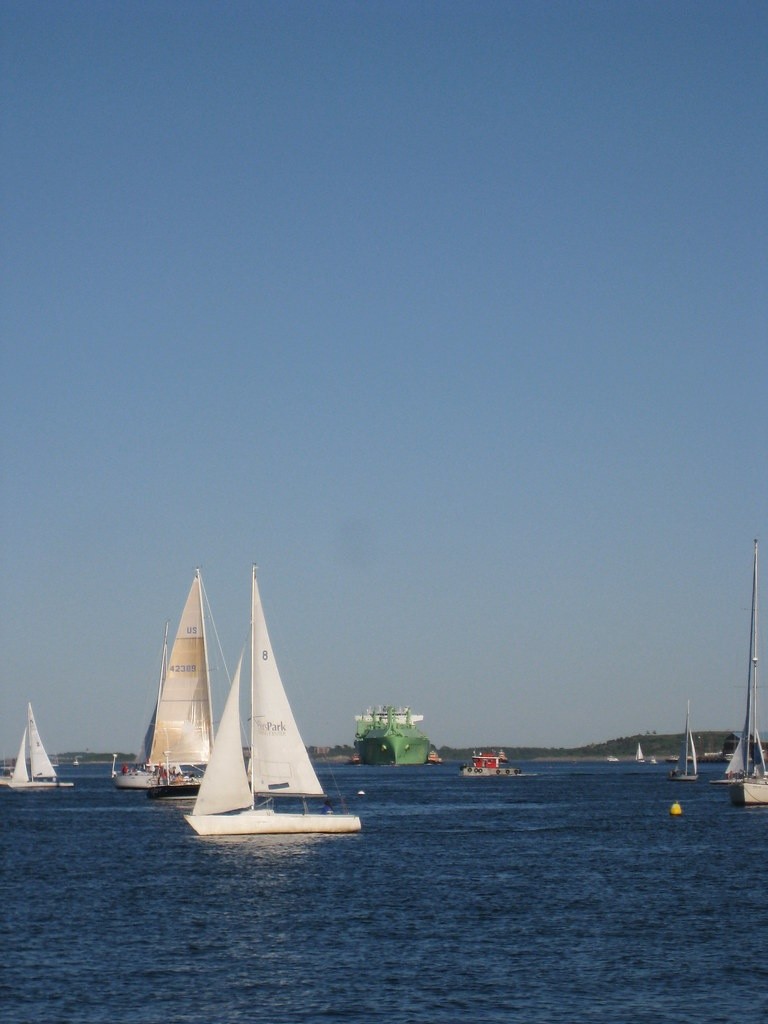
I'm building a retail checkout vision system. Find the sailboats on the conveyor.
[710,539,768,805]
[114,567,219,790]
[668,700,699,781]
[4,702,75,788]
[183,561,361,836]
[635,743,645,763]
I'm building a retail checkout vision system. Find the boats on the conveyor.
[147,750,201,801]
[665,755,679,761]
[429,751,442,764]
[650,757,657,764]
[354,705,429,766]
[460,747,521,776]
[350,753,360,764]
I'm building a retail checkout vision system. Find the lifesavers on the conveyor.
[496,770,500,774]
[474,768,483,773]
[514,770,518,774]
[468,768,472,772]
[506,770,510,774]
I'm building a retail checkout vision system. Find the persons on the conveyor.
[161,767,195,785]
[322,801,334,815]
[122,764,159,775]
[670,769,686,777]
